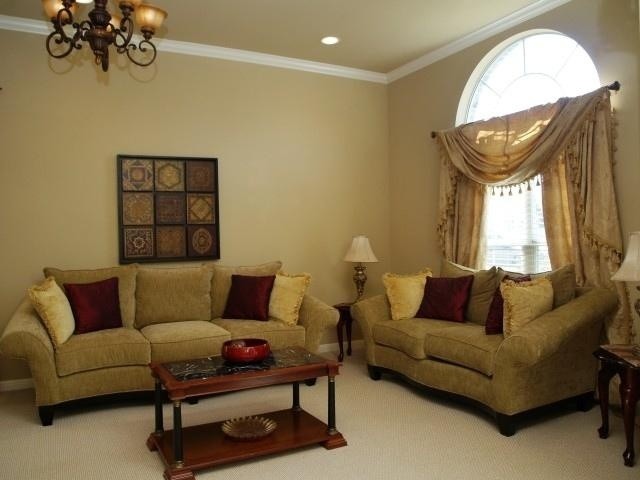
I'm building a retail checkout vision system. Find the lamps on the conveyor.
[41,0,170,75]
[608,231,640,319]
[343,235,380,303]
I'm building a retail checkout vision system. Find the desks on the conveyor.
[591,343,640,468]
[332,302,356,363]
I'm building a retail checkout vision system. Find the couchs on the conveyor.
[0,259,342,428]
[349,285,620,438]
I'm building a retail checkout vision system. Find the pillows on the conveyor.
[62,276,124,336]
[438,258,497,326]
[497,262,578,310]
[268,271,313,327]
[379,266,434,322]
[220,273,276,322]
[483,274,532,336]
[24,275,75,352]
[498,277,556,339]
[414,274,476,324]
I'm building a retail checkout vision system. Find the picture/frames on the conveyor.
[114,153,222,266]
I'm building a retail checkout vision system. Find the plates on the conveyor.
[220,416,278,440]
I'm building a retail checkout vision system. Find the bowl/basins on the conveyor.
[221,338,270,362]
